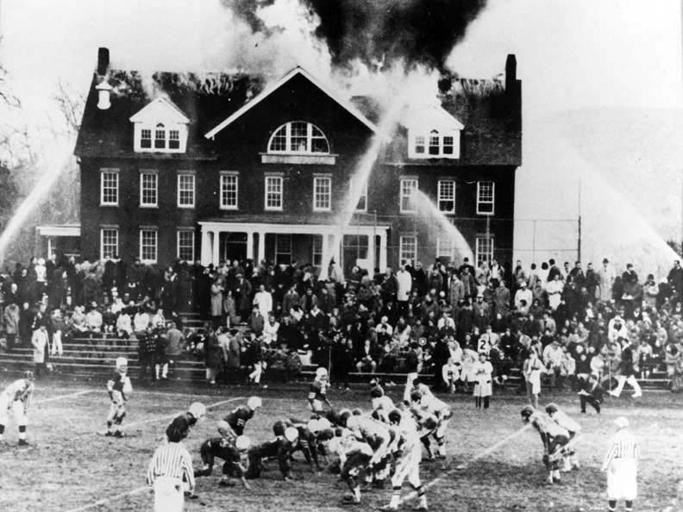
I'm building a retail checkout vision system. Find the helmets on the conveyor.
[247,396,262,410]
[369,386,386,397]
[116,357,128,372]
[23,371,37,381]
[188,402,207,420]
[410,390,422,402]
[316,368,328,381]
[546,403,558,414]
[235,408,401,450]
[521,407,533,417]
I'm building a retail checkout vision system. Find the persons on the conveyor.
[0,249,682,413]
[147,419,196,511]
[1,371,34,447]
[194,368,454,511]
[519,405,570,486]
[545,401,581,472]
[177,402,205,426]
[601,417,639,510]
[106,357,133,437]
